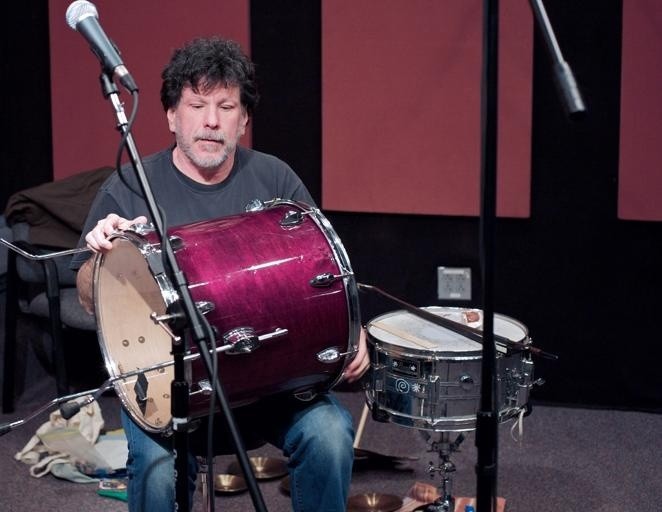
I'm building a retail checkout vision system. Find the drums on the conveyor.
[94,196,362,436]
[364,305,535,433]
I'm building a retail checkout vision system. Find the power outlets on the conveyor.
[435,265,474,302]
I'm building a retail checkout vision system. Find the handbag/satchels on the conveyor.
[13,395,105,478]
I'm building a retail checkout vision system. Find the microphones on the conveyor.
[64,0,139,95]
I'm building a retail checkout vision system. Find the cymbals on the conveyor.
[201,474,248,494]
[281,473,293,496]
[347,490,402,512]
[227,456,290,479]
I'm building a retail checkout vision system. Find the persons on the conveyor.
[69,38,372,512]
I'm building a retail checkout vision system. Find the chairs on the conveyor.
[173,405,273,512]
[1,166,116,415]
